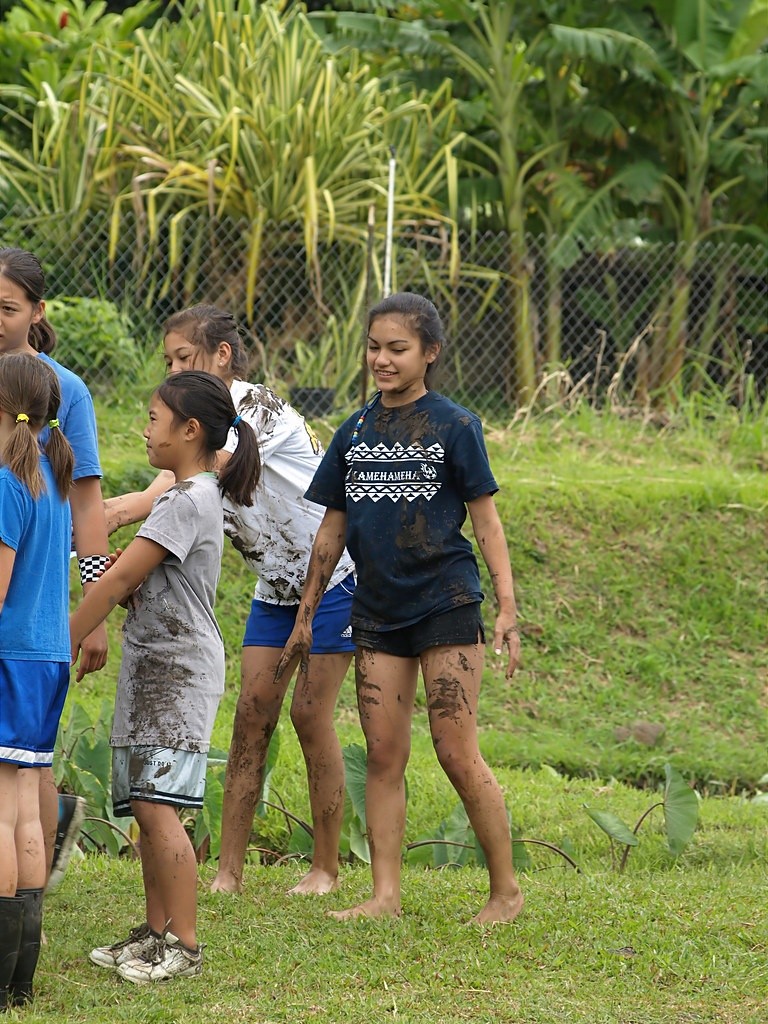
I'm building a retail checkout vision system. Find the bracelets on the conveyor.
[78,554,111,585]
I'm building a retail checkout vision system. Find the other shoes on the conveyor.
[43,794,86,894]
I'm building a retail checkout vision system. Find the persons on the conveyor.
[0,353,74,1014]
[277,292,524,926]
[102,305,356,896]
[65,368,262,983]
[1,246,109,682]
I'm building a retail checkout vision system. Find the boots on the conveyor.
[0,887,43,1013]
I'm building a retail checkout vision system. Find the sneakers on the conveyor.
[117,918,207,986]
[88,923,164,972]
